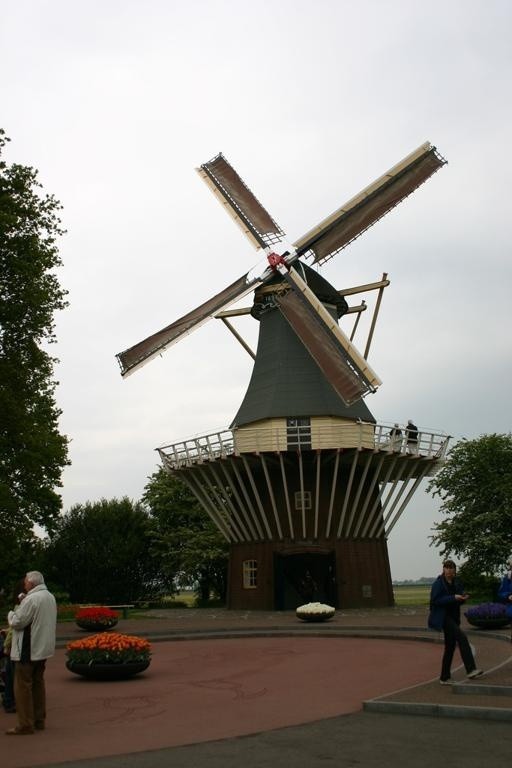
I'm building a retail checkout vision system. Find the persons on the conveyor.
[426,558,482,687]
[403,419,421,454]
[389,422,403,453]
[499,555,511,621]
[3,590,28,714]
[3,569,57,738]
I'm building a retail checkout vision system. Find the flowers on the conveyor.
[464,603,506,621]
[75,608,119,626]
[65,631,154,667]
[297,601,335,615]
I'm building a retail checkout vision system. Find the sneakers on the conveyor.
[466,667,484,679]
[439,679,456,686]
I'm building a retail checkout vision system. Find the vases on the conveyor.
[66,661,150,676]
[77,622,118,631]
[296,614,334,621]
[469,619,511,627]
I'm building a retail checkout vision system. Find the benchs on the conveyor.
[80,604,135,620]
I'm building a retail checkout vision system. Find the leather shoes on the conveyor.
[35,721,46,730]
[5,726,36,736]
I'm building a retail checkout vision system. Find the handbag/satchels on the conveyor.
[425,612,447,632]
